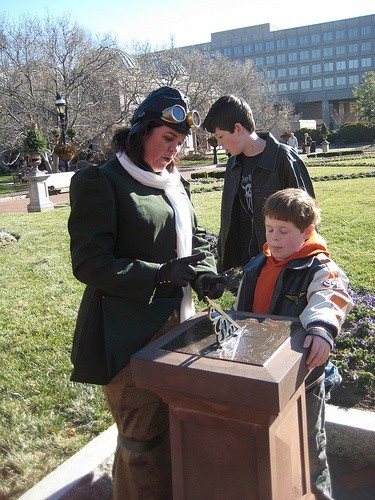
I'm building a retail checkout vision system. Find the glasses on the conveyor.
[131,104,201,129]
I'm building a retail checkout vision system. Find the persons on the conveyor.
[69,87,230,500]
[233,188,355,500]
[202,95,319,274]
[287,133,313,154]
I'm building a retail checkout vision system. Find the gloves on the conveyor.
[155,252,206,289]
[190,271,227,301]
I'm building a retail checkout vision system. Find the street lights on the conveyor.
[55,91,69,172]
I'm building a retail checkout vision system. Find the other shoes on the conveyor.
[325,373,342,403]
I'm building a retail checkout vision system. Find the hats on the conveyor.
[129,86,192,136]
